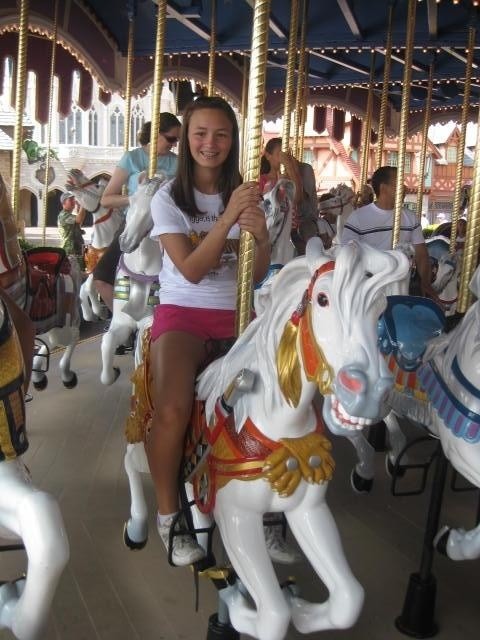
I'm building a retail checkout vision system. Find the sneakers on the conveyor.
[262,518,303,563]
[153,509,206,566]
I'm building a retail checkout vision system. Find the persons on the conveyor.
[146,97,273,566]
[461,208,468,221]
[290,157,319,242]
[258,137,305,257]
[58,192,89,328]
[437,213,448,225]
[421,210,429,229]
[367,178,374,202]
[344,164,444,306]
[91,113,182,355]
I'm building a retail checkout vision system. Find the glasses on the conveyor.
[160,132,180,144]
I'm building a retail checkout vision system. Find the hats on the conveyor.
[60,192,76,203]
